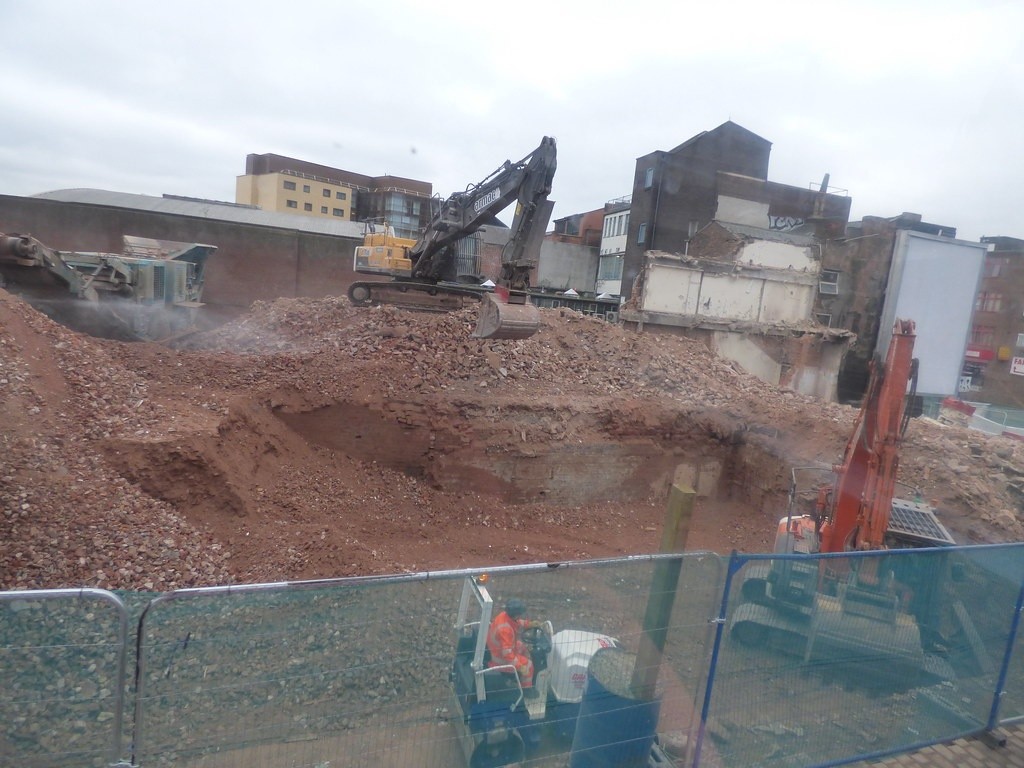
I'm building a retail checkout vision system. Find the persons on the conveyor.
[487,599,542,699]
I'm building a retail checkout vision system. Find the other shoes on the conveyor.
[521,688,539,698]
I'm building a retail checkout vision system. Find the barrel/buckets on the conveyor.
[571,647,664,768]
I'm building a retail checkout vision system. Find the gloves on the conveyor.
[527,620,543,631]
[518,665,531,677]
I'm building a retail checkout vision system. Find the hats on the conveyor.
[503,597,527,619]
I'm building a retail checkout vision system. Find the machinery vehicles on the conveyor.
[727,319,958,691]
[445,569,627,768]
[346,134,557,339]
[0,234,218,344]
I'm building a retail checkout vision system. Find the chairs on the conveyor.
[471,629,501,683]
